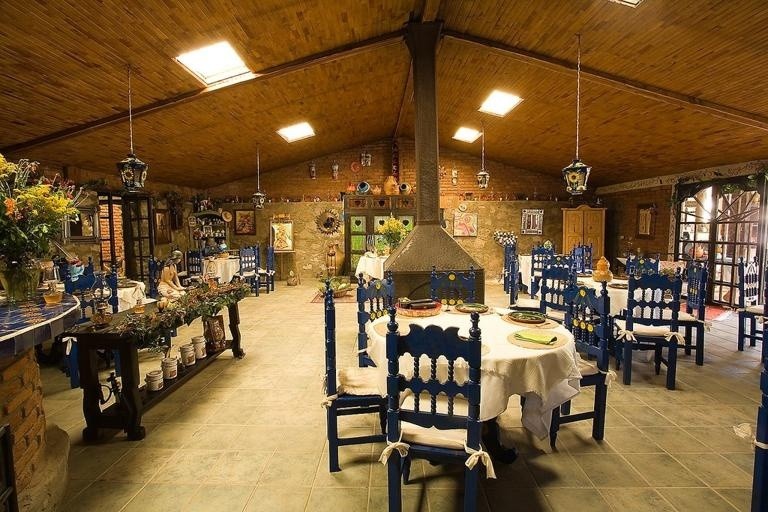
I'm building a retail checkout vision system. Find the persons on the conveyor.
[683,232,695,280]
[157,250,189,303]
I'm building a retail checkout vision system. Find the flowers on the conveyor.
[0,153,89,268]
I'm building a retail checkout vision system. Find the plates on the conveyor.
[456,300,488,314]
[609,283,628,288]
[510,310,546,323]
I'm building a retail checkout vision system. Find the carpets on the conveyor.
[311,283,382,303]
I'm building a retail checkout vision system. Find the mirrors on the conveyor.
[521,209,543,236]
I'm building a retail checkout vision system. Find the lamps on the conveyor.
[116,64,149,193]
[252,143,266,209]
[476,122,489,189]
[564,32,592,197]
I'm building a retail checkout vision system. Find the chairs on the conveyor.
[64,257,94,293]
[356,269,396,369]
[429,264,476,305]
[613,272,680,391]
[187,243,274,297]
[626,255,659,275]
[380,305,493,511]
[509,255,540,310]
[539,257,577,323]
[519,273,611,451]
[736,255,765,352]
[503,241,593,299]
[65,264,123,388]
[655,261,711,365]
[324,280,390,473]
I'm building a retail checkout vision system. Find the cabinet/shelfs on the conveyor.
[561,205,608,259]
[190,210,230,250]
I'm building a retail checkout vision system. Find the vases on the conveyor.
[0,257,42,303]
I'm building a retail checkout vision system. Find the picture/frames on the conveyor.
[270,219,296,253]
[235,209,256,235]
[635,204,656,241]
[154,208,171,244]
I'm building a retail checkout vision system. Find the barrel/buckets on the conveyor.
[145,335,207,391]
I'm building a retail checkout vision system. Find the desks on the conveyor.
[61,289,245,441]
[576,274,630,314]
[364,304,579,462]
